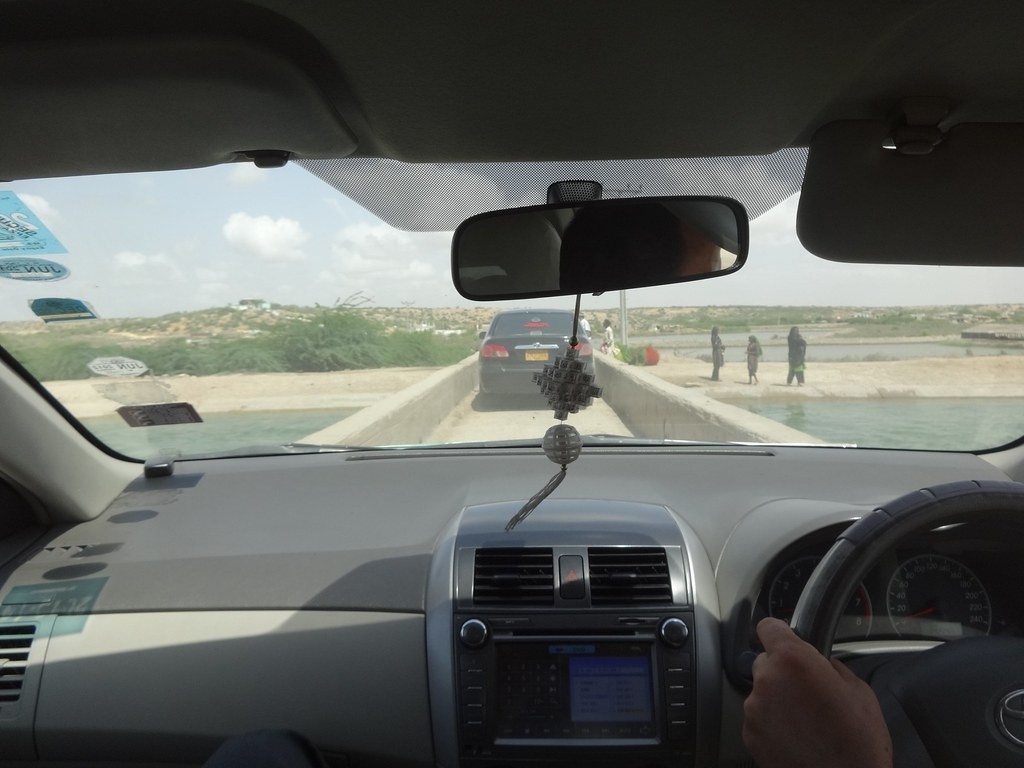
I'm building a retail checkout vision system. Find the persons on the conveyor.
[576,315,620,359]
[711,327,729,378]
[786,328,805,385]
[744,336,764,383]
[738,619,893,768]
[558,199,722,291]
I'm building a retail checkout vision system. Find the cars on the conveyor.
[478,308,596,397]
